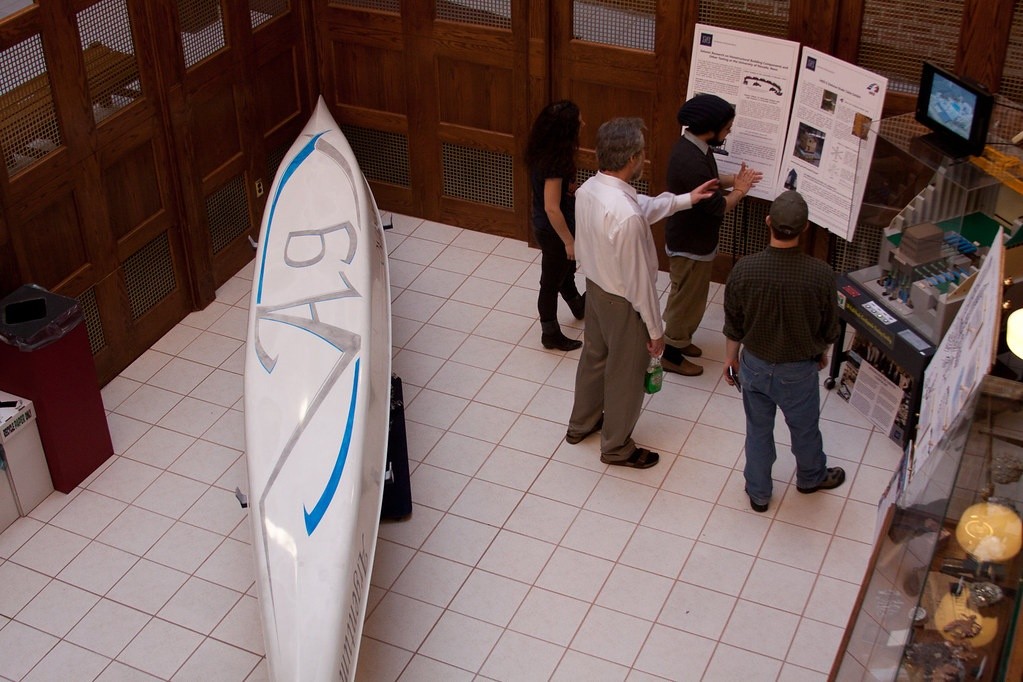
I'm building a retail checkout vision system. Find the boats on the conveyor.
[233,96,397,682]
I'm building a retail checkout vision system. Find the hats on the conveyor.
[677,95,735,134]
[769,191,808,235]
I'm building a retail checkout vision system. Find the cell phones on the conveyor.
[728,366,741,393]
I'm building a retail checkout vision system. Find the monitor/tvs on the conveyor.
[914,60,994,160]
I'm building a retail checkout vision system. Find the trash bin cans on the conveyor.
[0,283,114,495]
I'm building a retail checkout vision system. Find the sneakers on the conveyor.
[660,355,703,376]
[797,467,846,494]
[671,344,701,356]
[749,497,768,513]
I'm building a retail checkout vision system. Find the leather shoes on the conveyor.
[577,291,586,320]
[541,332,582,352]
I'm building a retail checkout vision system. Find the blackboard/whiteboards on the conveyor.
[902,226,1004,489]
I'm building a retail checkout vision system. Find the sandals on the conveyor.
[565,413,604,444]
[600,448,660,468]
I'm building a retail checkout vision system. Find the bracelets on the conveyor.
[732,189,746,196]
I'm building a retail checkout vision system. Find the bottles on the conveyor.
[644,350,664,395]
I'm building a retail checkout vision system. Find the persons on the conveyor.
[660,90,764,375]
[564,115,723,470]
[723,191,844,512]
[520,101,587,352]
